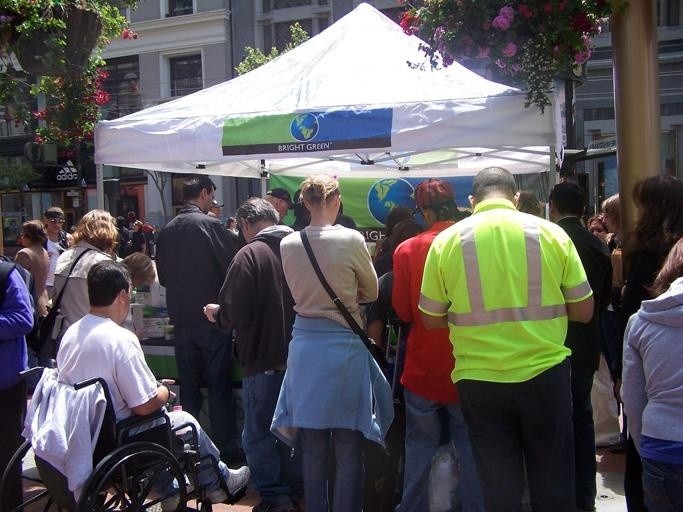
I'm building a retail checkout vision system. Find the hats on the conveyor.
[411,180,452,215]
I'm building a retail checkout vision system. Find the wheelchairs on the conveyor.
[0,364,248,512]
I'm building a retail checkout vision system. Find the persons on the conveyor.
[587,214,610,241]
[0,258,36,512]
[417,166,595,509]
[42,209,116,367]
[14,220,55,395]
[619,237,683,512]
[278,177,377,510]
[548,181,615,512]
[392,177,489,512]
[203,198,307,512]
[56,259,251,508]
[263,187,294,227]
[290,188,312,232]
[519,192,544,220]
[613,175,683,401]
[364,218,428,500]
[209,199,224,219]
[600,193,626,455]
[152,175,241,468]
[225,217,239,236]
[41,206,73,299]
[108,212,159,289]
[371,206,413,263]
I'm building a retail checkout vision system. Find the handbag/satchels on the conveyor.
[27,244,90,352]
[300,229,392,379]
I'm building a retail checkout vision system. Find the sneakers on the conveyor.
[207,465,252,503]
[161,482,194,511]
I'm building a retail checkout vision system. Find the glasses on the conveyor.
[588,226,606,236]
[50,216,67,227]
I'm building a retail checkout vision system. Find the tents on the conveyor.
[87,4,562,234]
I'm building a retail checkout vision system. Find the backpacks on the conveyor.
[0,256,40,344]
